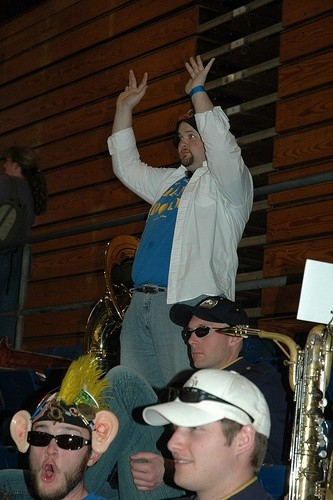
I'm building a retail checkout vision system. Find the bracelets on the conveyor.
[188,86,204,99]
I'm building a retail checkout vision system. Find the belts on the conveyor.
[136,286,166,294]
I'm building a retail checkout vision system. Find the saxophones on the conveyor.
[209,319,333,500]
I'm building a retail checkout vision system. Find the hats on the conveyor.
[142,370,271,439]
[32,351,118,433]
[169,295,250,326]
[175,107,199,143]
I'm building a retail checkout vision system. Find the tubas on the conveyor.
[83,233,140,376]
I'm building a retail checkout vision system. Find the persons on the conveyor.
[0,145,48,249]
[107,55,255,390]
[0,297,294,500]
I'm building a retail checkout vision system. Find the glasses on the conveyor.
[162,386,254,423]
[182,326,226,341]
[27,431,92,451]
